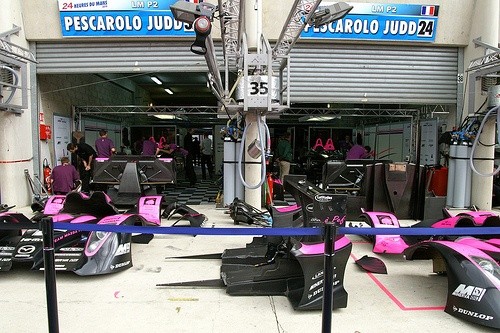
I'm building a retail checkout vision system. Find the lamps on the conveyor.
[308,2,354,28]
[150,76,164,86]
[170,0,219,24]
[247,139,262,159]
[164,88,174,94]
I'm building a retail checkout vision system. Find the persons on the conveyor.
[193,137,201,166]
[158,132,168,149]
[184,128,194,169]
[134,137,145,152]
[348,144,371,160]
[67,143,97,193]
[200,133,213,179]
[95,129,114,158]
[159,140,197,185]
[274,132,292,184]
[147,135,160,147]
[52,157,79,195]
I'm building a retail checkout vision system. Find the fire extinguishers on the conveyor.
[267,173,277,204]
[45,166,53,195]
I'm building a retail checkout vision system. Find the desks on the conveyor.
[92,181,174,197]
